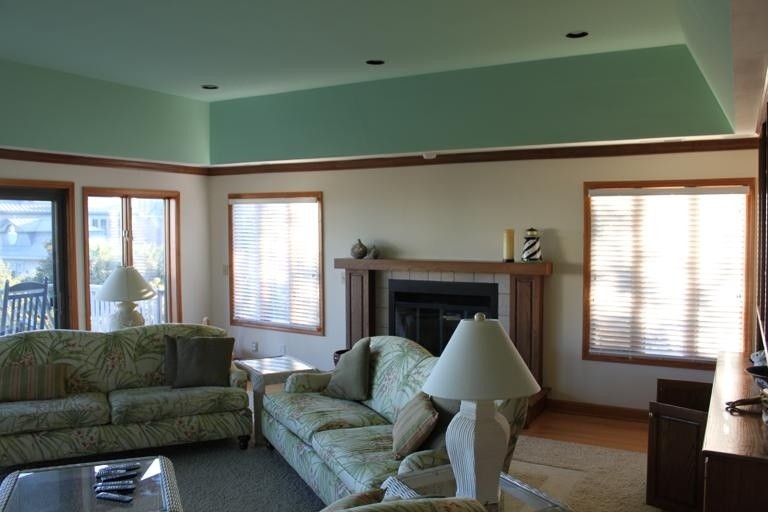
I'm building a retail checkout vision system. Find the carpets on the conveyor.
[12,389,648,512]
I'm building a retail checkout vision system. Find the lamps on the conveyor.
[95,266,157,329]
[421,311,541,506]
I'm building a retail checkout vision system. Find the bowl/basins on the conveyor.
[744,365,768,389]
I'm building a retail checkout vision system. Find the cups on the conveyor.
[502,228,514,263]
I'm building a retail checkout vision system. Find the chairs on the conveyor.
[0,277,48,333]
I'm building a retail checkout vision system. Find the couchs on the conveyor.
[261,335,528,508]
[0,323,251,467]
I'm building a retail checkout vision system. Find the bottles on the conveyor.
[521,227,541,263]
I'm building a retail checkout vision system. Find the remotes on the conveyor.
[101,471,137,481]
[96,491,134,503]
[96,480,133,485]
[95,485,137,491]
[100,462,141,471]
[95,470,126,478]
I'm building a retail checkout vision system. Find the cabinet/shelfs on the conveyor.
[647,348,768,512]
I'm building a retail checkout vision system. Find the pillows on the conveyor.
[392,391,439,461]
[163,334,177,386]
[0,364,66,400]
[171,337,234,388]
[321,337,371,400]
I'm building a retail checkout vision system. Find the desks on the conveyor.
[233,355,318,447]
[380,462,575,512]
